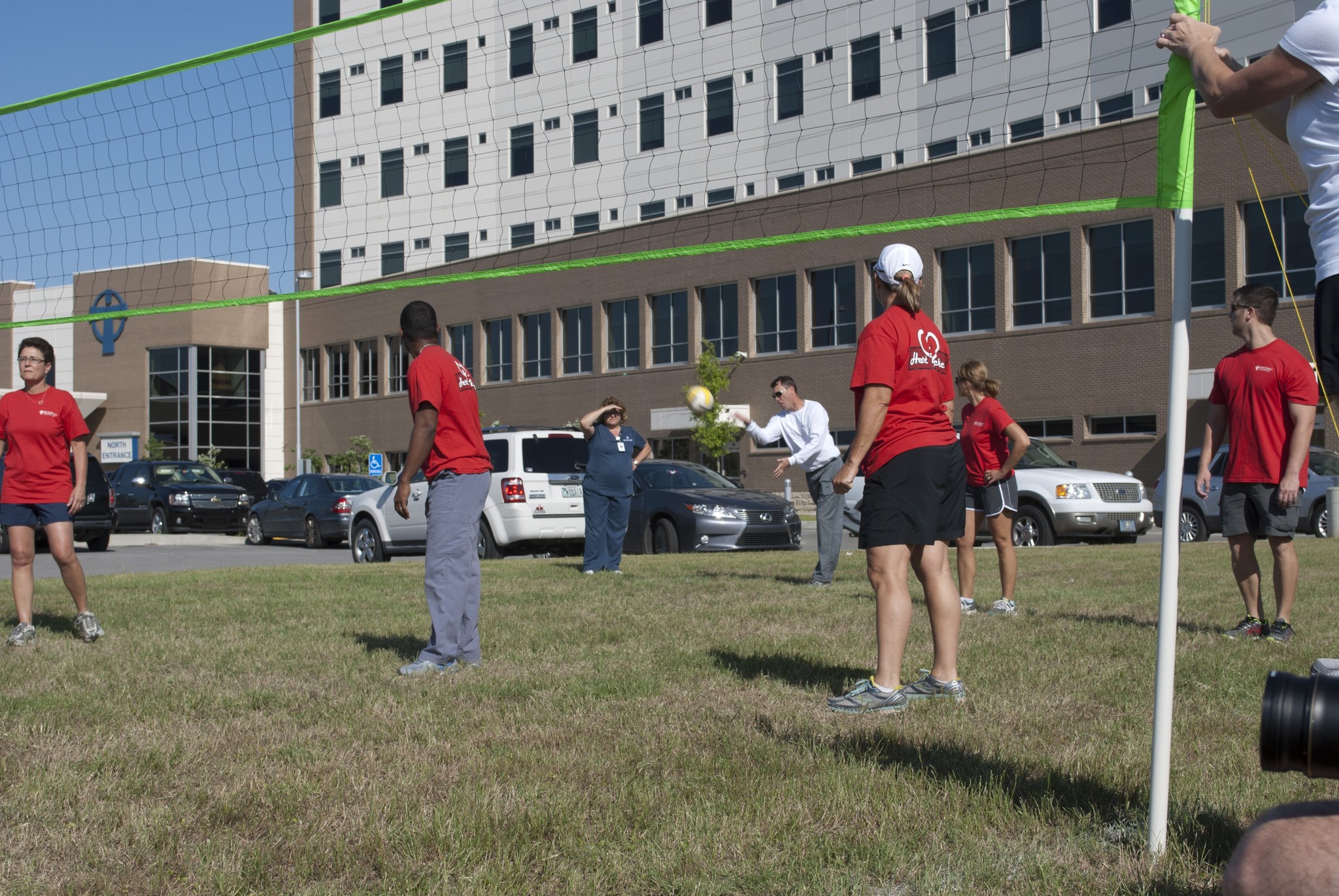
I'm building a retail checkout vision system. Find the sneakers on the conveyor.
[901,669,967,702]
[73,611,105,642]
[986,598,1018,617]
[960,598,977,614]
[397,657,457,679]
[1223,613,1271,642]
[5,622,36,649]
[1265,618,1298,645]
[825,679,909,714]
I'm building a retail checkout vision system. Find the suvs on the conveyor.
[108,457,250,537]
[347,424,590,564]
[0,450,118,555]
[840,429,1153,547]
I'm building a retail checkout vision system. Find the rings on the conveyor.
[987,477,990,480]
[398,508,402,510]
[1162,29,1169,37]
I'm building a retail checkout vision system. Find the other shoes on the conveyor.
[1309,657,1338,678]
[808,577,831,587]
[613,570,622,575]
[583,569,595,575]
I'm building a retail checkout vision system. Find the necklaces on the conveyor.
[25,384,48,405]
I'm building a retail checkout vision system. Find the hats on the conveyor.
[873,243,923,285]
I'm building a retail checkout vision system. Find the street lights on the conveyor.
[294,270,314,478]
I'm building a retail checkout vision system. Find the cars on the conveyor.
[264,478,289,502]
[1152,444,1339,543]
[213,468,268,506]
[246,473,389,548]
[622,458,802,556]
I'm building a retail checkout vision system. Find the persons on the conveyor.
[0,338,104,648]
[827,243,969,716]
[734,375,863,589]
[1220,799,1339,896]
[956,357,1030,615]
[1155,0,1339,677]
[580,397,651,574]
[394,300,495,677]
[1196,284,1319,648]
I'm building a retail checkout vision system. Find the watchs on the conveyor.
[632,459,639,466]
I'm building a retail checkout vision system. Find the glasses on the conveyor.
[772,387,788,398]
[954,377,962,385]
[17,356,46,365]
[1230,303,1260,313]
